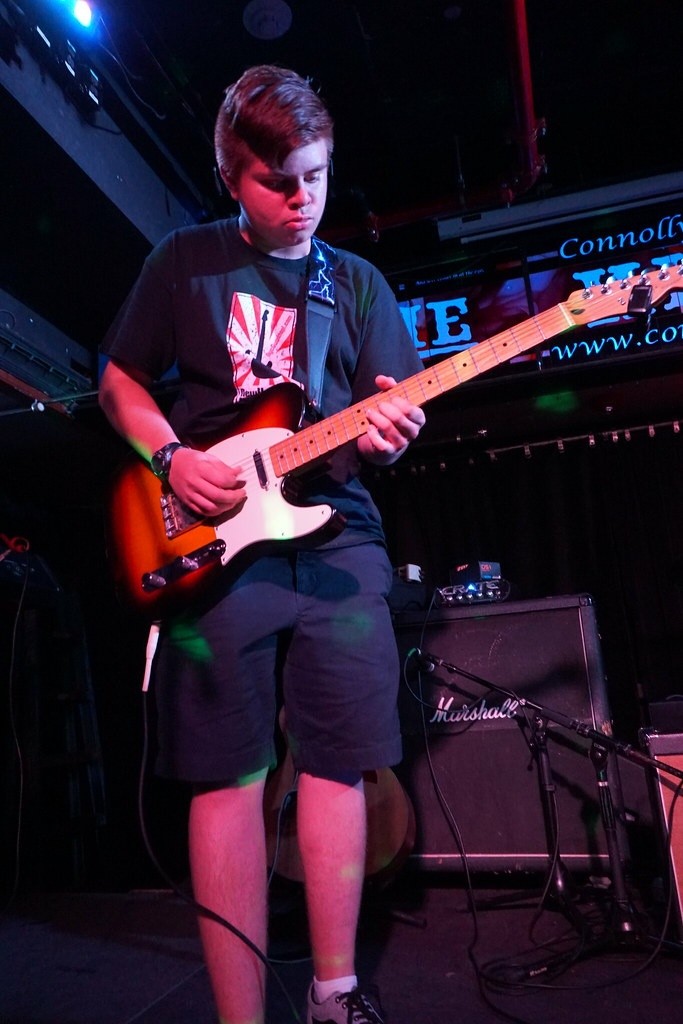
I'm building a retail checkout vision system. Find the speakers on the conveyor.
[642,729,683,919]
[397,597,643,882]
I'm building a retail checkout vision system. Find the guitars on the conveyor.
[273,705,419,886]
[101,258,682,637]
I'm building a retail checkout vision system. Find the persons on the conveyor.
[99,66,426,1024]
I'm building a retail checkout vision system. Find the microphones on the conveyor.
[412,648,435,672]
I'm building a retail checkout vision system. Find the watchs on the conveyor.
[150,442,186,480]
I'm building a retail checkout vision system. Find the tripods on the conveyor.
[414,657,682,984]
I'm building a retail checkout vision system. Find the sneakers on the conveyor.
[306,982,384,1024]
